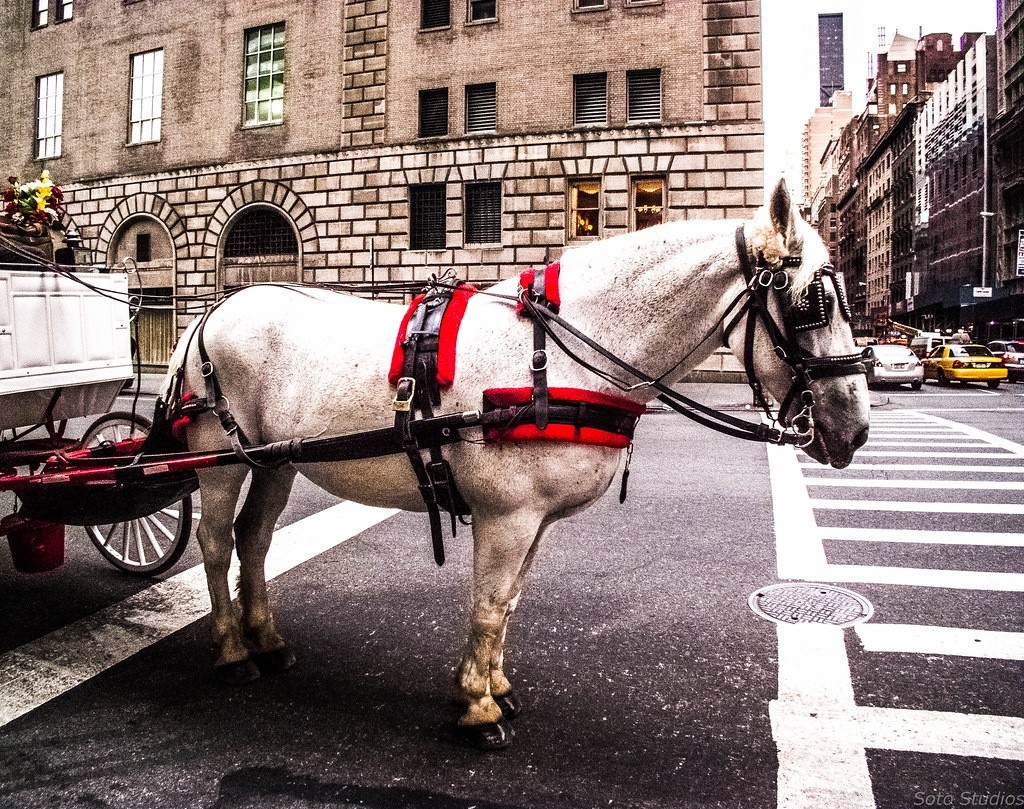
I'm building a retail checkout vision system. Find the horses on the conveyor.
[155,179,871,723]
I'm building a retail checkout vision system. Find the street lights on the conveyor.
[858,281,890,343]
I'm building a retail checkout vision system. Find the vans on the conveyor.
[908,336,954,359]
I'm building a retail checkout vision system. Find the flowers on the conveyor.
[4,168,65,229]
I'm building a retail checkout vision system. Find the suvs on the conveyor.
[986,340,1024,380]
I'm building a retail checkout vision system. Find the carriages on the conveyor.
[1,172,874,752]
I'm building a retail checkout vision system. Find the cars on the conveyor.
[921,344,1009,389]
[861,344,924,391]
